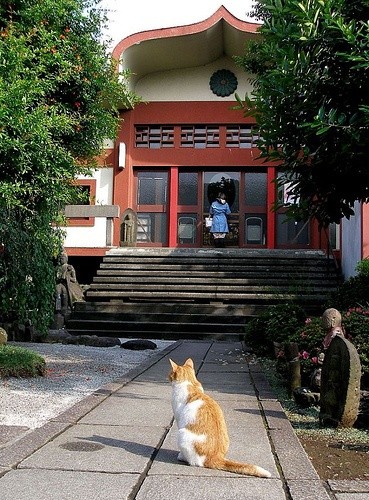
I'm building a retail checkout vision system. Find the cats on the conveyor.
[167,357,272,478]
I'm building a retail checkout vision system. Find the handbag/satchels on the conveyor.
[205,214,213,226]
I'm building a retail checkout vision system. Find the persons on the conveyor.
[209,192,231,247]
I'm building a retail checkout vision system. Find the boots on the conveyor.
[214,238,219,248]
[219,238,225,248]
